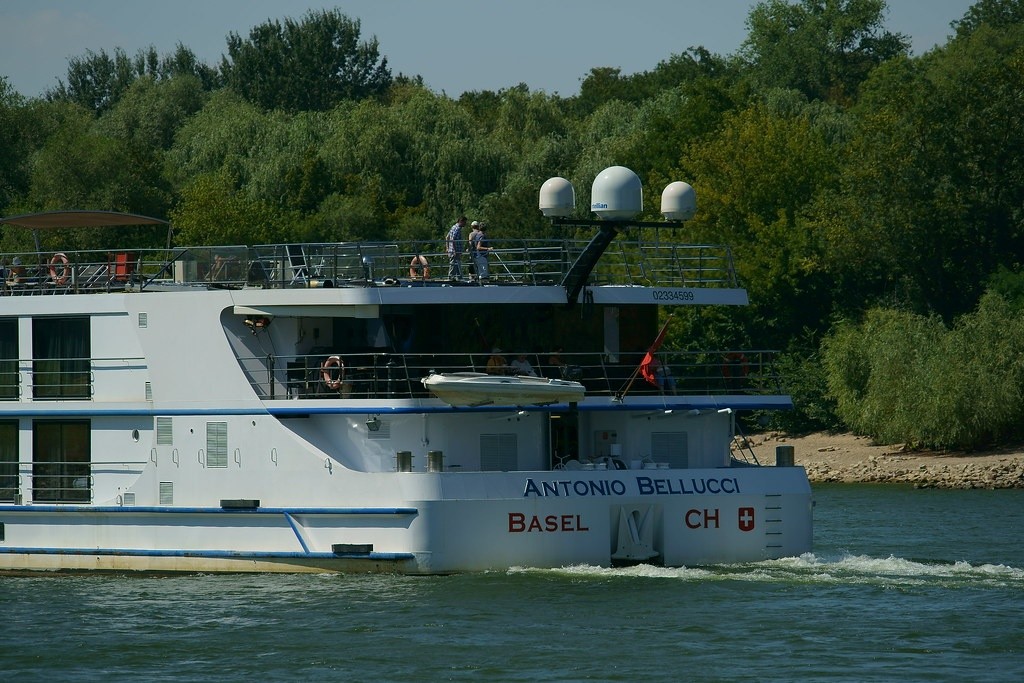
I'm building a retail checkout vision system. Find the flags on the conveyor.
[640,318,673,386]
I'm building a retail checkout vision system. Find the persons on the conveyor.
[445,217,489,283]
[488,346,585,381]
[6,256,53,283]
[208,254,226,277]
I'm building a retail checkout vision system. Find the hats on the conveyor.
[551,344,561,352]
[471,221,479,226]
[479,222,486,230]
[491,347,503,353]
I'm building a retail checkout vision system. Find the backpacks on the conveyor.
[727,354,745,376]
[468,230,479,250]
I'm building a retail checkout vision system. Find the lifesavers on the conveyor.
[324,356,344,389]
[50,253,69,285]
[409,256,430,279]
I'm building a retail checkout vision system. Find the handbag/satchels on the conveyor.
[656,366,671,377]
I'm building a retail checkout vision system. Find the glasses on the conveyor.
[556,350,562,353]
[461,220,466,223]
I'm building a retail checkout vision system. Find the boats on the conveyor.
[419,368,585,410]
[0,163,818,579]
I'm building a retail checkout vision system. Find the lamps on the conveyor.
[365,415,382,432]
